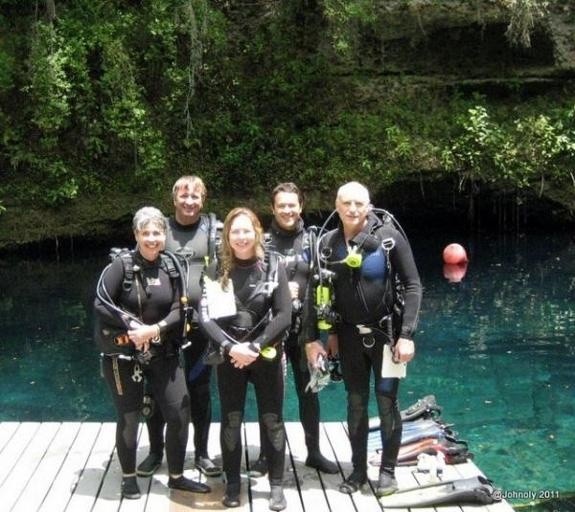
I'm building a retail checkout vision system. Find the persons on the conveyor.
[196,203,292,511]
[262,182,340,478]
[301,181,424,498]
[134,174,229,476]
[93,205,212,499]
